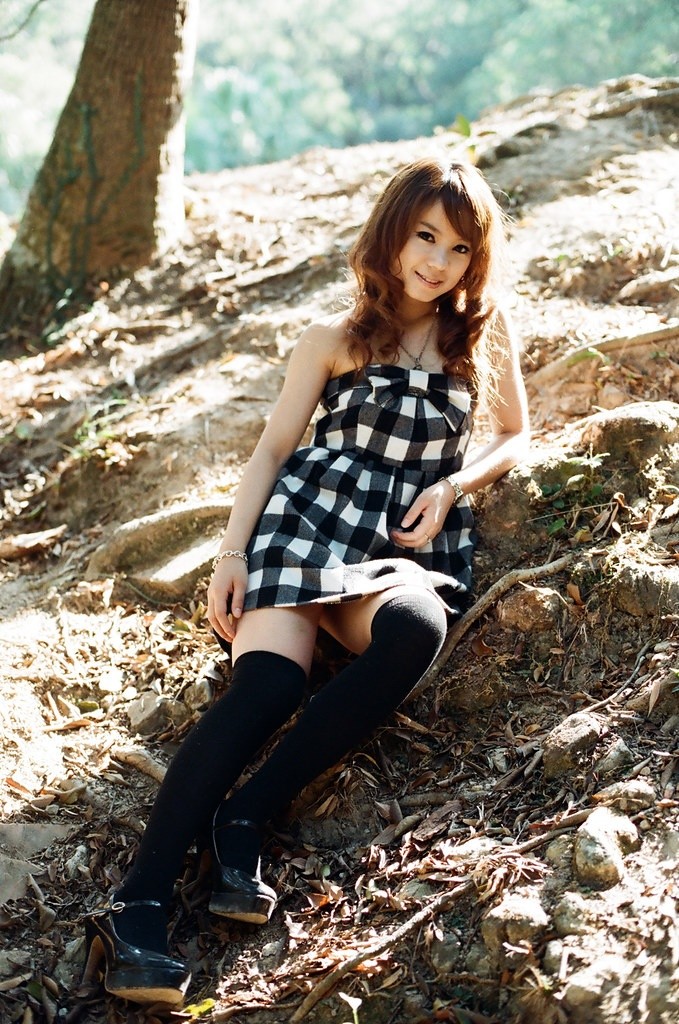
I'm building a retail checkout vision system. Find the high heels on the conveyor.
[81,890,192,1004]
[196,799,277,923]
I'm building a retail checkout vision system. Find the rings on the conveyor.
[425,535,432,544]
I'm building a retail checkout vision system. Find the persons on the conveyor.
[77,154,533,1001]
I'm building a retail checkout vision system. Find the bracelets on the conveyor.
[210,551,249,569]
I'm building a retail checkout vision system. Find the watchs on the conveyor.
[440,475,463,506]
